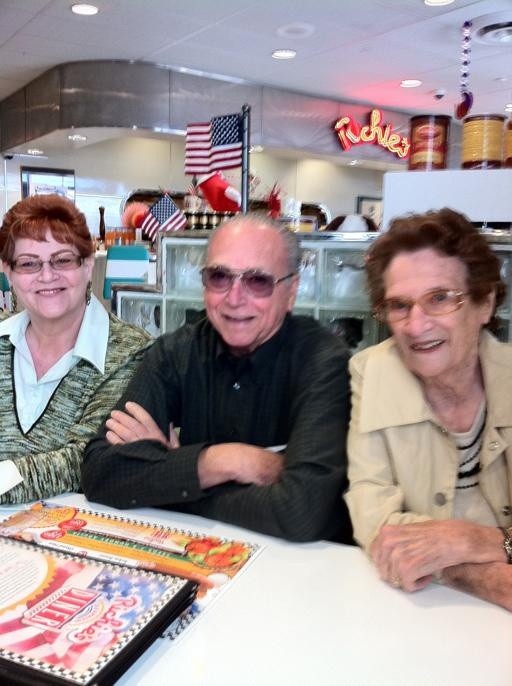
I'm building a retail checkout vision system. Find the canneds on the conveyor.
[181,210,241,230]
[408,114,512,169]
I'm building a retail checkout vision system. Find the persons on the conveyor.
[79,210,363,548]
[0,192,157,508]
[338,206,512,616]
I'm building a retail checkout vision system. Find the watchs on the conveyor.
[498,525,511,564]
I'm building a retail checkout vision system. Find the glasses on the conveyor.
[211,270,295,297]
[371,284,479,325]
[8,250,87,274]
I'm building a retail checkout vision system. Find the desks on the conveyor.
[0,493,512,686]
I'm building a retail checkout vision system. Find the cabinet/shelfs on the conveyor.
[116,230,512,355]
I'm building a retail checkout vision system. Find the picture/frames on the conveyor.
[357,195,383,228]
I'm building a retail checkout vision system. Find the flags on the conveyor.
[184,112,244,175]
[140,194,190,245]
[187,177,199,195]
